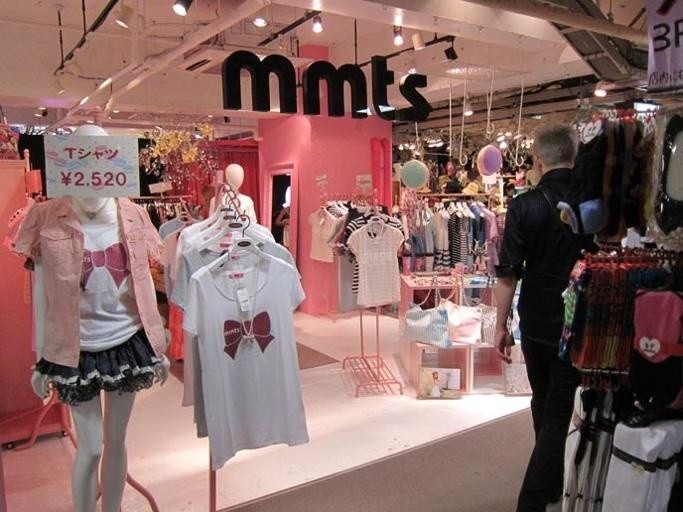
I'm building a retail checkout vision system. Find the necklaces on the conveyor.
[74,199,111,219]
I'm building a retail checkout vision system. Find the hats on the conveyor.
[437,169,480,194]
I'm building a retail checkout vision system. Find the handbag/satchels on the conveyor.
[401,300,514,347]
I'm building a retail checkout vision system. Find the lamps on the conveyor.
[35,105,49,119]
[594,80,608,98]
[392,25,403,46]
[399,49,418,75]
[52,79,67,95]
[253,4,269,27]
[312,14,323,34]
[411,32,427,52]
[173,2,194,17]
[443,34,460,63]
[113,1,134,29]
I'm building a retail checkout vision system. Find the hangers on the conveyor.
[319,193,386,229]
[417,194,477,209]
[134,183,271,272]
[584,246,678,266]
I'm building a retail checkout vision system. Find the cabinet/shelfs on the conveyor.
[165,140,261,225]
[0,160,65,446]
[398,273,503,393]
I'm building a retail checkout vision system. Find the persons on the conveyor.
[276,187,290,249]
[439,159,482,193]
[492,121,602,512]
[503,182,514,207]
[9,125,171,512]
[210,163,261,219]
[429,372,442,397]
